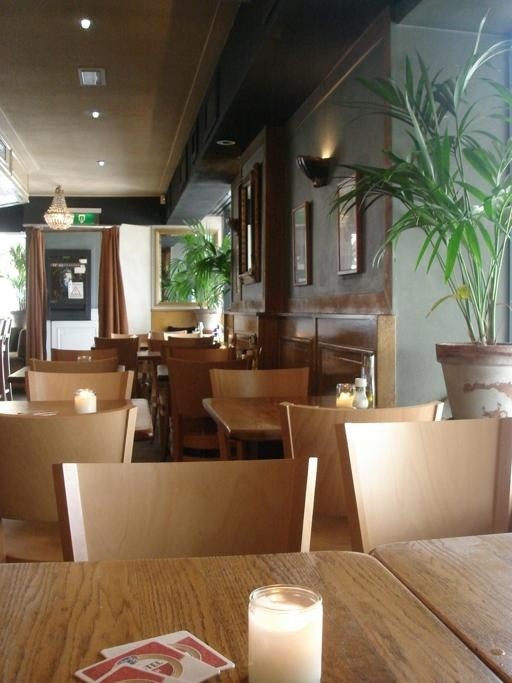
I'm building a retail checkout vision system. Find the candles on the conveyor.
[74,390,98,413]
[335,383,355,409]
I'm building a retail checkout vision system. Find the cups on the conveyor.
[198,321,204,337]
[335,382,354,407]
[74,387,97,413]
[355,377,369,407]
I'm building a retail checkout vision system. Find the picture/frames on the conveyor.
[336,167,363,276]
[291,201,313,288]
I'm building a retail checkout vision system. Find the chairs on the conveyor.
[169,336,214,348]
[282,398,446,552]
[337,416,512,555]
[0,408,138,562]
[95,338,139,370]
[24,369,136,399]
[163,331,187,339]
[148,339,169,351]
[210,369,310,461]
[50,348,118,361]
[167,357,253,461]
[50,454,321,563]
[161,347,237,365]
[30,358,119,372]
[112,332,150,344]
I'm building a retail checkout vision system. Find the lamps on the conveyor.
[43,187,75,231]
[297,150,332,186]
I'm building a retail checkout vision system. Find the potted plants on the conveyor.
[327,8,511,419]
[8,243,27,327]
[163,220,234,334]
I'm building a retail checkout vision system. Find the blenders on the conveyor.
[233,348,250,369]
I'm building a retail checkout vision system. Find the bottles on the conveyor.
[361,352,375,408]
[352,377,369,409]
